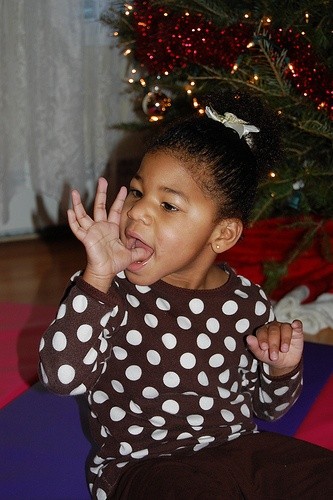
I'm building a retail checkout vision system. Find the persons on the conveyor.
[42,89,333,500]
